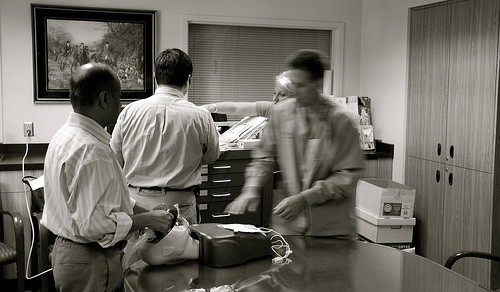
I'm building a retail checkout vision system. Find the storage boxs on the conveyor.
[356,235,415,255]
[355,178,416,218]
[355,207,415,243]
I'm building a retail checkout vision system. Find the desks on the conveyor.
[124,235,494,292]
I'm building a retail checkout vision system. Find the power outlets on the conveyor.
[23,122,34,136]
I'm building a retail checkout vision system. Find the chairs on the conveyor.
[29,187,55,292]
[0,184,25,292]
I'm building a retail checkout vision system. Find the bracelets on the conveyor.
[211,103,218,113]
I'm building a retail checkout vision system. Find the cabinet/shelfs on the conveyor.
[197,149,273,228]
[405,2,500,290]
[0,169,49,277]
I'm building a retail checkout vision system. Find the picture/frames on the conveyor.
[30,2,156,105]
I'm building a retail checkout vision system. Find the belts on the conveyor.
[128,184,194,192]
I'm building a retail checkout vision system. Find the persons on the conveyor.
[224,50,365,238]
[43,63,174,292]
[200,69,296,123]
[109,47,221,225]
